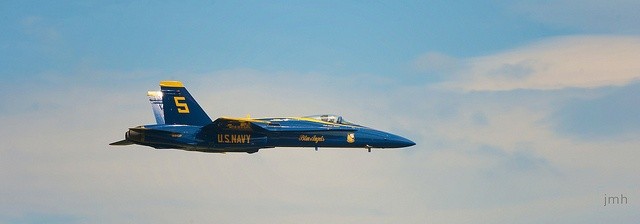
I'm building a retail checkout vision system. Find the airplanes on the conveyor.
[109,80,416,153]
